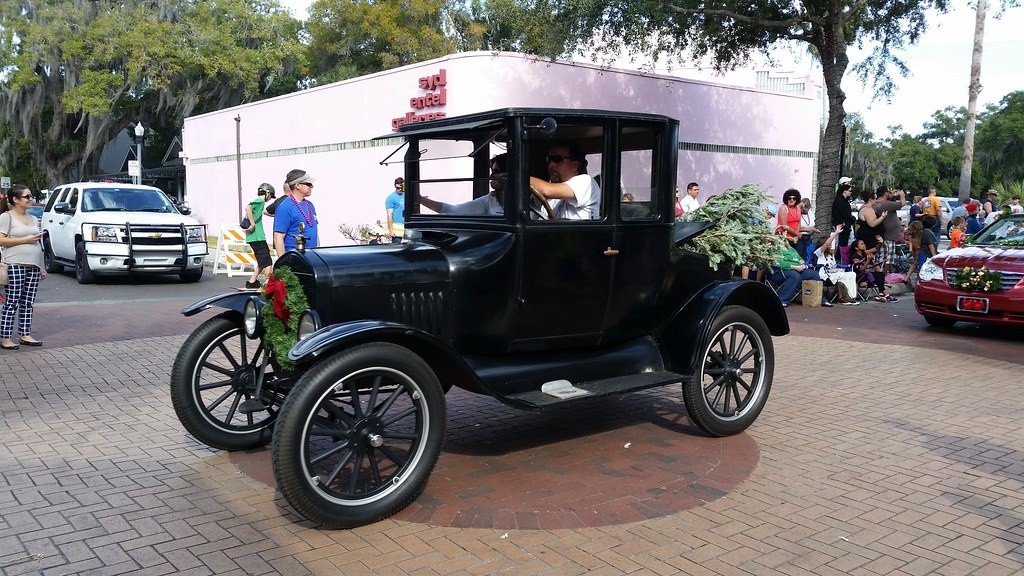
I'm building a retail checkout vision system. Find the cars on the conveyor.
[896,200,912,233]
[851,200,864,211]
[170,108,791,530]
[7,203,47,230]
[41,182,210,284]
[912,197,980,240]
[914,213,1024,328]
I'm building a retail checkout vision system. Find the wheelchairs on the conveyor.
[895,244,915,270]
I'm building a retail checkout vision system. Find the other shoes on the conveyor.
[782,303,788,307]
[0,342,19,350]
[19,338,43,346]
[823,302,833,307]
[837,300,860,305]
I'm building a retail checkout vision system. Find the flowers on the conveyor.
[948,264,1003,293]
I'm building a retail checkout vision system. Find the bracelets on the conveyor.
[882,216,885,219]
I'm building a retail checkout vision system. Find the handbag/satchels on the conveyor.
[0,261,9,286]
[240,218,251,228]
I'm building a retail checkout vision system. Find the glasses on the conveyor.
[300,183,312,186]
[675,191,678,193]
[546,155,571,163]
[19,194,33,200]
[788,196,797,201]
[395,186,402,189]
[492,168,507,174]
[849,190,853,192]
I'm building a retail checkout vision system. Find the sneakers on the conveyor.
[245,280,262,289]
[875,294,899,303]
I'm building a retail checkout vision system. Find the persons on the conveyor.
[385,177,406,242]
[0,185,48,348]
[242,169,321,287]
[529,137,602,220]
[419,152,541,218]
[623,176,1024,307]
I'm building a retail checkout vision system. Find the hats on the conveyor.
[986,189,998,195]
[288,173,317,184]
[839,177,853,185]
[966,204,978,213]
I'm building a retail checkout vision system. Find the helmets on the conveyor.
[258,183,276,198]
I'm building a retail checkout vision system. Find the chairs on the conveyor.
[619,203,650,218]
[764,243,881,304]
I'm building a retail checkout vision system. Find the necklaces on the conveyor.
[924,227,939,243]
[290,192,314,228]
[800,213,811,226]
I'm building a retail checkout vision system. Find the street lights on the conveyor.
[134,120,145,185]
